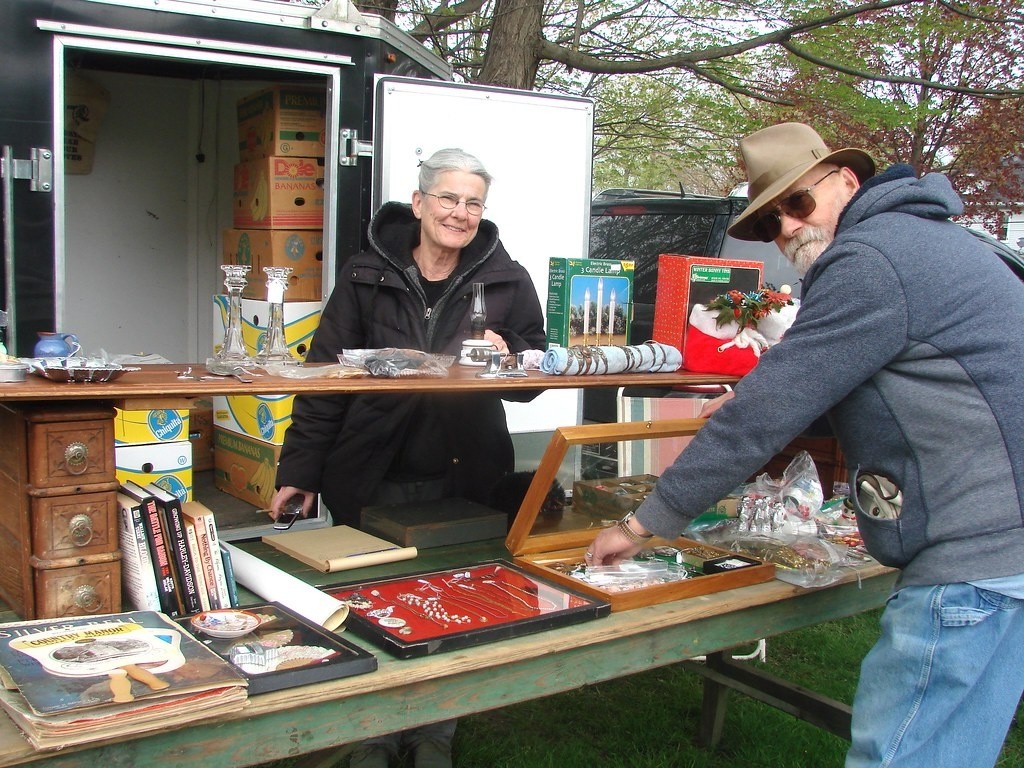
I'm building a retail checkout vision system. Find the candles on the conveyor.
[596,278,603,333]
[609,288,615,334]
[584,288,590,334]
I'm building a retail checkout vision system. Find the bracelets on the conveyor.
[561,340,667,375]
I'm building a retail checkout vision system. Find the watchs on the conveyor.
[618,511,651,545]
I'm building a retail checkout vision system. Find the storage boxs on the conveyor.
[212,394,297,445]
[219,227,322,302]
[312,556,612,660]
[572,473,661,523]
[212,294,323,364]
[652,254,764,372]
[544,256,635,352]
[114,441,192,506]
[212,425,283,513]
[359,496,508,548]
[506,418,778,613]
[235,85,326,162]
[112,396,197,444]
[172,602,379,696]
[233,158,324,230]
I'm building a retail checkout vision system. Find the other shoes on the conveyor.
[414,731,452,768]
[683,304,769,376]
[758,297,801,347]
[349,743,389,768]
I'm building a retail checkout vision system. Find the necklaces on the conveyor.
[371,571,557,629]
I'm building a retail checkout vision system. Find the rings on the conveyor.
[494,341,503,350]
[585,550,593,558]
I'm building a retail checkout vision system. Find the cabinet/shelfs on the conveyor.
[0,364,903,768]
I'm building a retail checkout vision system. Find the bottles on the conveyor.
[253,267,301,367]
[205,264,254,374]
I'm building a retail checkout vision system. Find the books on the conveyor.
[0,610,250,753]
[261,524,418,573]
[117,480,240,619]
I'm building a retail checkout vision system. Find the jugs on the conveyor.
[34,332,81,358]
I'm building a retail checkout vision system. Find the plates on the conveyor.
[429,353,457,367]
[190,609,261,638]
[31,364,141,382]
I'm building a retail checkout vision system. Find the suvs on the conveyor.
[581,182,806,425]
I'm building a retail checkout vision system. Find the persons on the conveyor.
[584,122,1024,768]
[270,147,548,768]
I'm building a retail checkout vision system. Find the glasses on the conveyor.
[421,191,488,216]
[752,169,841,244]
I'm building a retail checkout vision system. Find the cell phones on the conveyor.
[273,496,305,530]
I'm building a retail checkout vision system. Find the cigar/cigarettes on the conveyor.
[256,506,287,514]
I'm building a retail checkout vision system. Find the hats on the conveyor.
[727,122,876,242]
